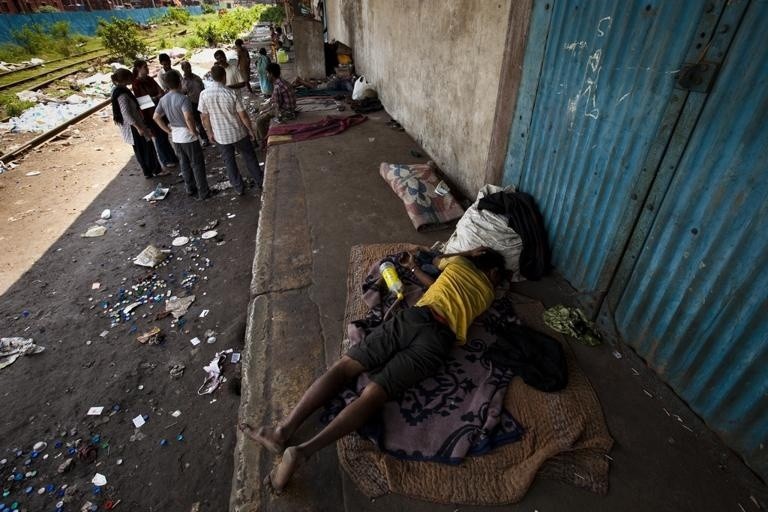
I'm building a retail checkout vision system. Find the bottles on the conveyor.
[380,261,405,299]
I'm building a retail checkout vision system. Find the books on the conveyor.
[135,94,156,111]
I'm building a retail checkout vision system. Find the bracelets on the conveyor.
[411,266,419,272]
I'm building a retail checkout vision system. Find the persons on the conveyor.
[269,25,290,62]
[255,62,297,150]
[133,60,179,168]
[152,70,218,199]
[215,51,257,155]
[197,66,263,196]
[181,61,209,146]
[110,69,171,178]
[157,54,183,94]
[257,49,273,99]
[235,40,253,93]
[239,245,508,490]
